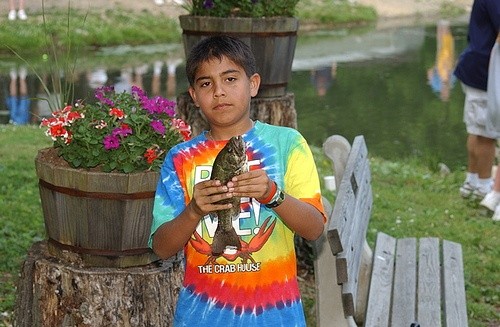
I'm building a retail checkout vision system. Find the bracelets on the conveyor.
[255,180,277,204]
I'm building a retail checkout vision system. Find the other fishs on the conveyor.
[208,134,247,257]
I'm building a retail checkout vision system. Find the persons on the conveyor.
[450,0,500,200]
[8,69,39,126]
[148,36,327,327]
[88,65,110,91]
[8,0,27,20]
[153,57,182,103]
[35,70,62,124]
[121,63,152,95]
[307,42,338,96]
[479,33,500,225]
[154,0,184,6]
[426,16,459,102]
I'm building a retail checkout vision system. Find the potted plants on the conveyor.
[177,0,299,99]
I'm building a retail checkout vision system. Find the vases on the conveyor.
[34,148,162,268]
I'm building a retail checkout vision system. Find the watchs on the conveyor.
[266,187,286,207]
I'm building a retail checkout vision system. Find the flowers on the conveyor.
[0,0,193,173]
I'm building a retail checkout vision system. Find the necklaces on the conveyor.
[208,129,215,140]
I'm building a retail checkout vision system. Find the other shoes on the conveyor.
[480,194,498,211]
[472,187,487,200]
[460,181,476,198]
[491,209,500,222]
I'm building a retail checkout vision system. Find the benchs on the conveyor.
[313,134,468,327]
